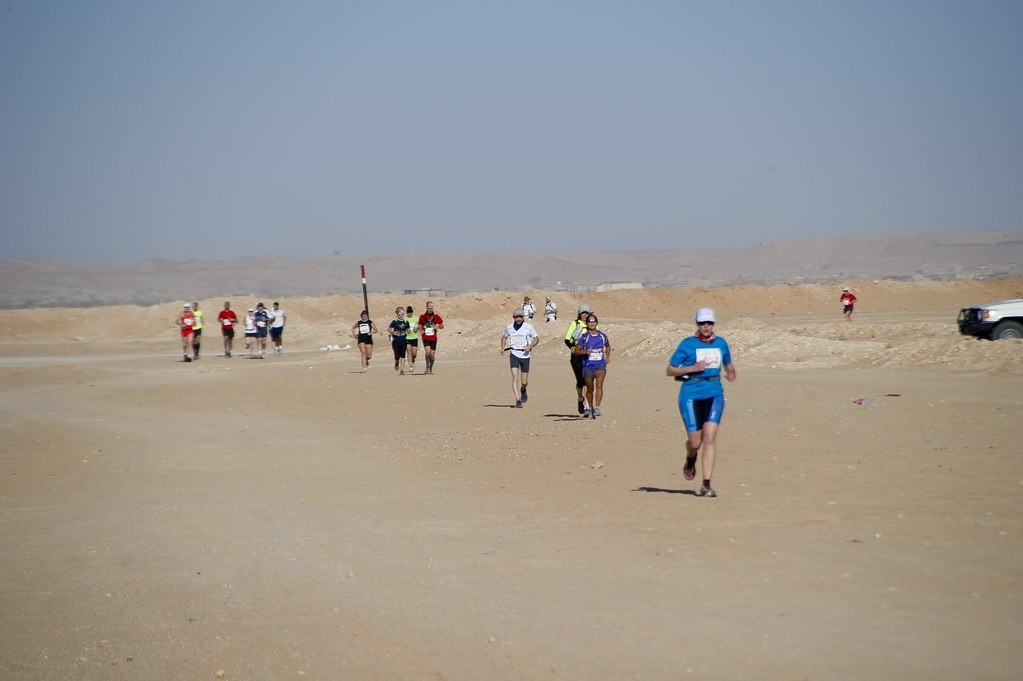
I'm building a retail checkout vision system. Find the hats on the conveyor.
[546,295,551,300]
[695,308,715,322]
[524,297,531,301]
[513,308,524,317]
[406,306,413,311]
[426,301,433,307]
[578,304,591,313]
[183,302,191,307]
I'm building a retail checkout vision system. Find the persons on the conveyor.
[242,302,286,359]
[217,302,237,356]
[175,302,204,362]
[521,294,558,322]
[666,308,736,497]
[388,301,444,375]
[840,289,857,323]
[564,304,610,419]
[501,308,539,408]
[351,311,378,373]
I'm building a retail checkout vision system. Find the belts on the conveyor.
[504,347,531,351]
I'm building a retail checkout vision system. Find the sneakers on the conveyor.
[701,486,717,497]
[683,451,697,480]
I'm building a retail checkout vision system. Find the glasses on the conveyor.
[587,319,596,322]
[696,321,714,325]
[581,311,588,314]
[407,311,413,313]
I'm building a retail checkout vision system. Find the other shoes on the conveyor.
[578,396,585,414]
[360,366,368,373]
[224,350,232,359]
[521,387,528,403]
[393,364,433,375]
[516,401,522,408]
[245,345,282,359]
[583,406,601,419]
[183,353,199,362]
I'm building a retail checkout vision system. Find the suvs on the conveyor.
[957,299,1023,341]
